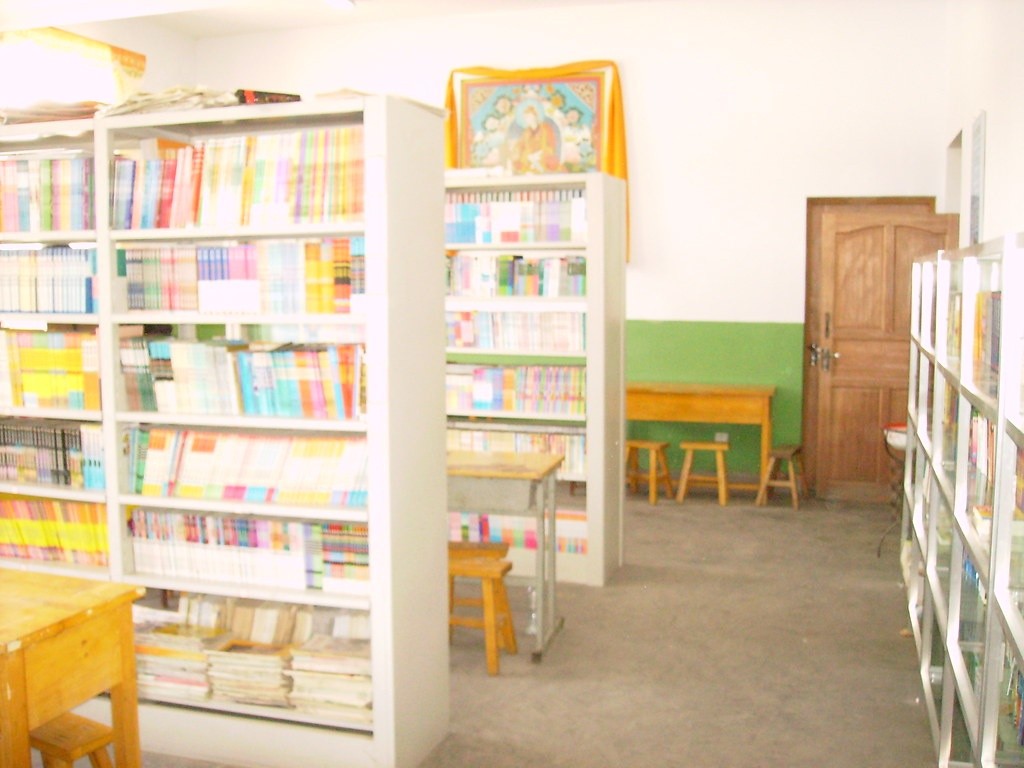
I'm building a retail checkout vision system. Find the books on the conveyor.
[943,288,1024,749]
[0,128,588,720]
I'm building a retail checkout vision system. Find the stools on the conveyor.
[449,540,519,674]
[754,445,809,511]
[676,441,731,507]
[624,439,675,503]
[28,711,114,768]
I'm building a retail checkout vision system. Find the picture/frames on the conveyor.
[453,62,615,172]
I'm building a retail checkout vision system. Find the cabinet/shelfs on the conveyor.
[901,230,1024,768]
[443,173,626,590]
[1,94,450,768]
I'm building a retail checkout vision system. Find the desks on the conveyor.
[625,382,778,509]
[445,450,565,662]
[0,566,146,768]
